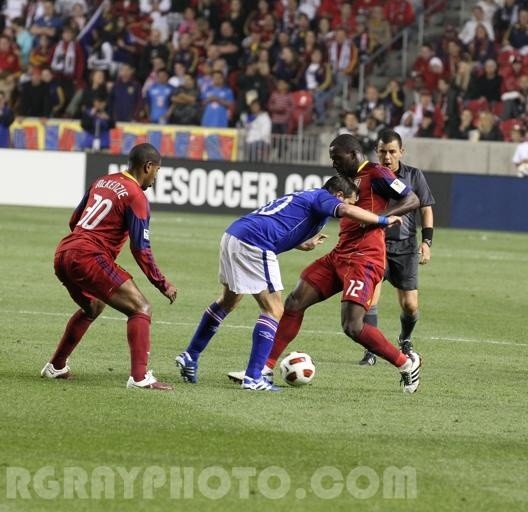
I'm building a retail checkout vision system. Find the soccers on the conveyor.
[279,351,315,385]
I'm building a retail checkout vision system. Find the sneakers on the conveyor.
[40,362,78,380]
[359,349,376,366]
[399,350,422,395]
[396,334,414,357]
[227,363,282,391]
[126,370,173,391]
[175,351,198,385]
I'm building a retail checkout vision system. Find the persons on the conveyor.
[174,172,404,392]
[336,1,528,178]
[358,129,437,366]
[227,134,423,394]
[1,1,392,165]
[41,144,177,391]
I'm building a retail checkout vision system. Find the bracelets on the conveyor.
[421,227,434,248]
[378,217,389,225]
